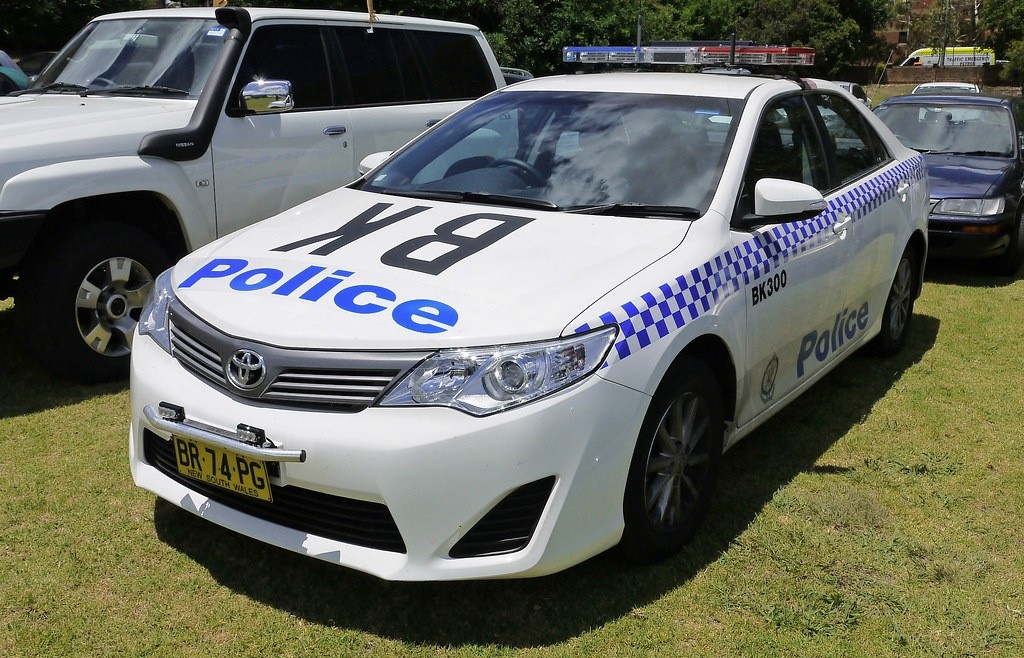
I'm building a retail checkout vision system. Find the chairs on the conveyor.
[900,111,1008,151]
[558,112,798,211]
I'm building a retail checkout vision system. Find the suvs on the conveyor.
[0,8,536,385]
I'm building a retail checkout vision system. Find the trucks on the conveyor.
[900,46,995,67]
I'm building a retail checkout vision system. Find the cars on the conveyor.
[698,81,1024,278]
[129,50,931,582]
